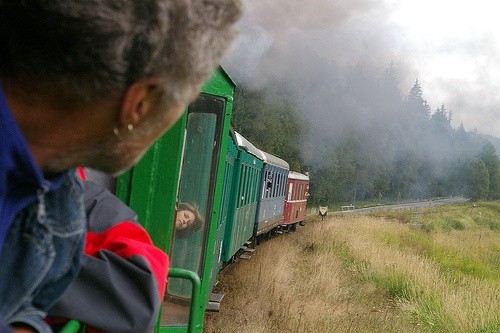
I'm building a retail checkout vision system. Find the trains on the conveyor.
[59,62,311,333]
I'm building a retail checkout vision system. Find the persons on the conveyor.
[176,202,203,239]
[267,175,272,192]
[0,0,243,333]
